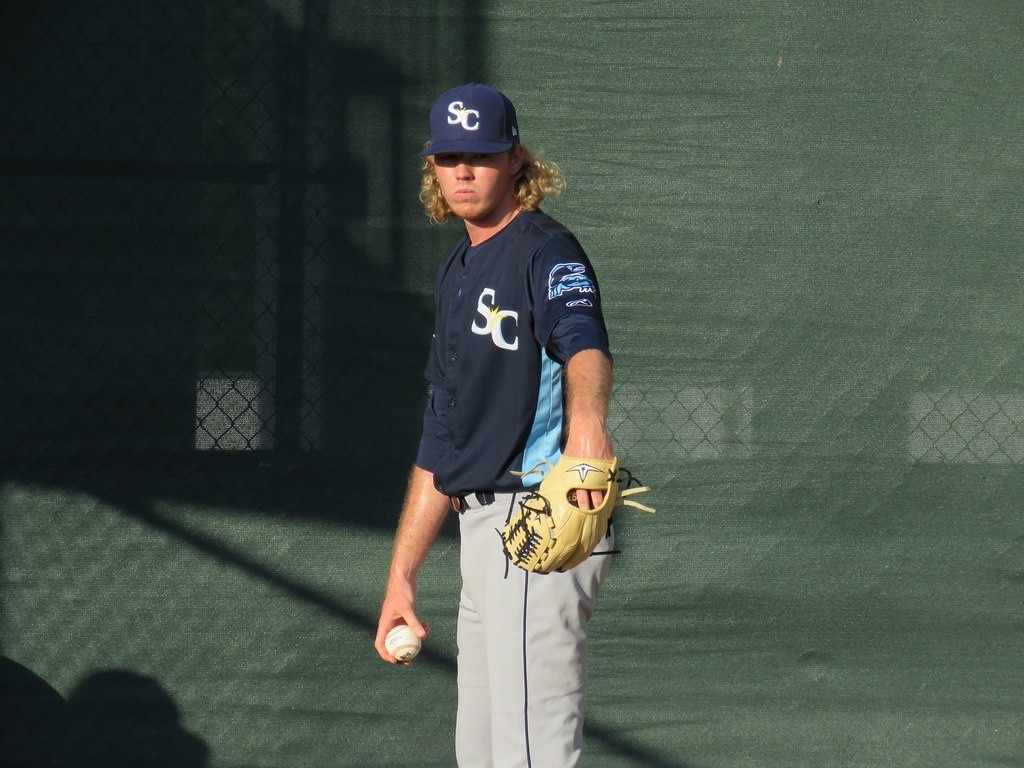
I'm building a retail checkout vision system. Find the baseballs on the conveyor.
[384,625,422,661]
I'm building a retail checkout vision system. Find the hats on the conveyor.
[418,82,520,155]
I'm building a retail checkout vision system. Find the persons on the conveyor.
[373,83,619,768]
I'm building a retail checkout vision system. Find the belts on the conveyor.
[450,491,496,514]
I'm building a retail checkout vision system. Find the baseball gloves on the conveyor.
[500,455,620,576]
